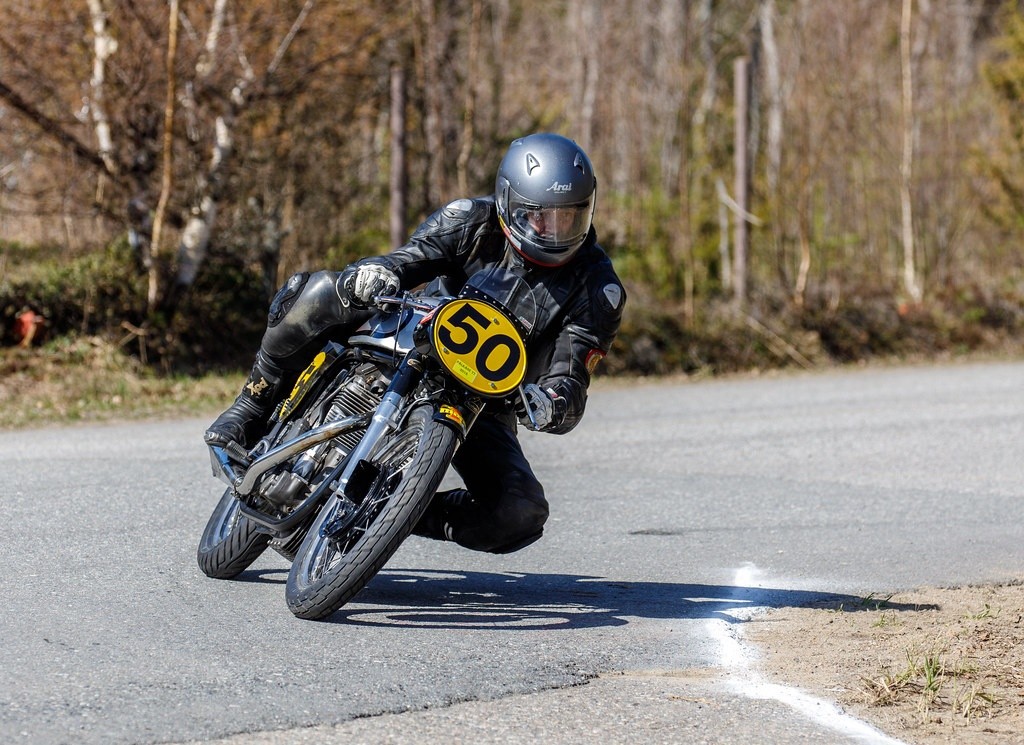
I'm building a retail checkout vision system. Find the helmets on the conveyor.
[495,132,596,269]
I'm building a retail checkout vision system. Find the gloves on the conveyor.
[334,261,401,314]
[515,383,567,432]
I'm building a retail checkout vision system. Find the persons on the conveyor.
[203,130,627,554]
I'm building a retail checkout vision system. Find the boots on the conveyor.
[204,349,303,449]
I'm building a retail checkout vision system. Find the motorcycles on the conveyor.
[195,266,538,622]
